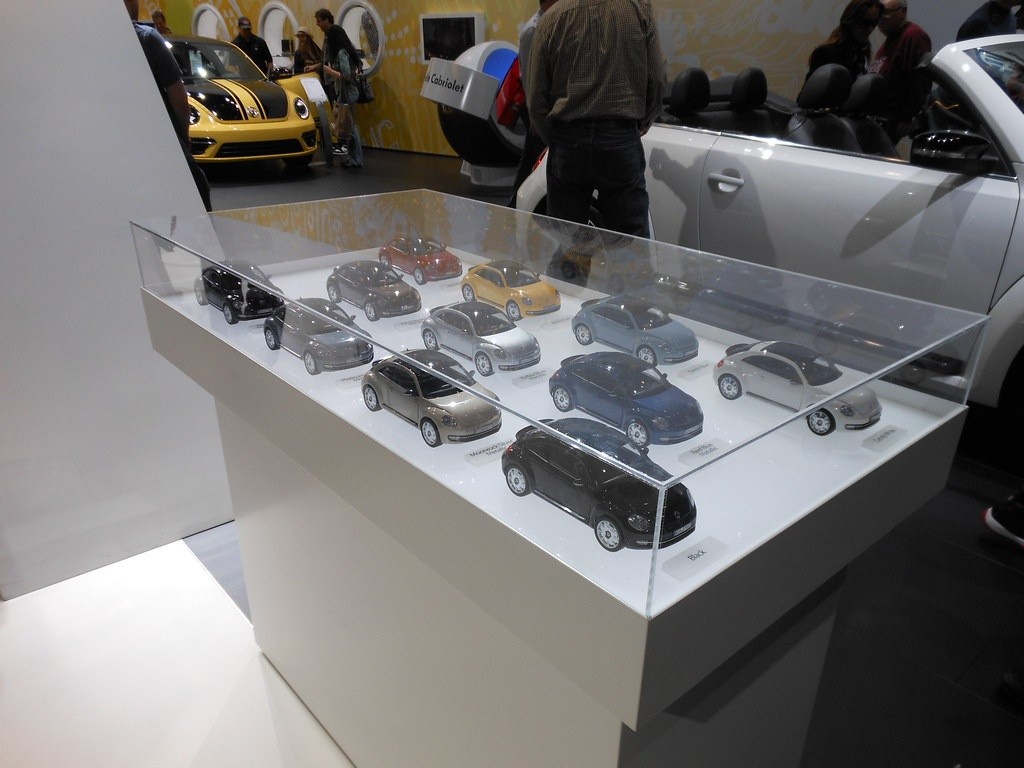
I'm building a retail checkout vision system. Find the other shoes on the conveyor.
[983,493,1024,545]
[341,160,361,169]
[333,146,348,154]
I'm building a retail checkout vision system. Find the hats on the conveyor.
[293,27,314,39]
[238,16,251,30]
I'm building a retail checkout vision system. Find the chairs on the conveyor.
[786,64,864,154]
[837,74,903,159]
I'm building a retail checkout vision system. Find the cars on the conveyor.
[501,417,698,553]
[324,260,422,322]
[163,33,333,173]
[459,259,561,321]
[264,296,376,374]
[713,339,883,436]
[379,235,464,285]
[361,345,503,448]
[548,351,704,446]
[193,258,286,326]
[421,302,541,377]
[572,293,699,366]
[516,31,1024,478]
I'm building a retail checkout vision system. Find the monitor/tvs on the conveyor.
[420,12,485,65]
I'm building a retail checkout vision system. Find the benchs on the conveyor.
[656,66,782,139]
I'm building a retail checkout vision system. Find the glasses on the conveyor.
[883,6,905,16]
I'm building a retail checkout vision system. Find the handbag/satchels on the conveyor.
[355,62,375,104]
[490,54,526,129]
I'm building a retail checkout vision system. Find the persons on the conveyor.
[796,0,885,103]
[123,0,212,213]
[324,25,363,167]
[294,27,322,75]
[152,11,172,33]
[956,0,1024,132]
[304,9,348,155]
[528,0,691,303]
[508,0,558,208]
[229,17,273,77]
[867,0,931,154]
